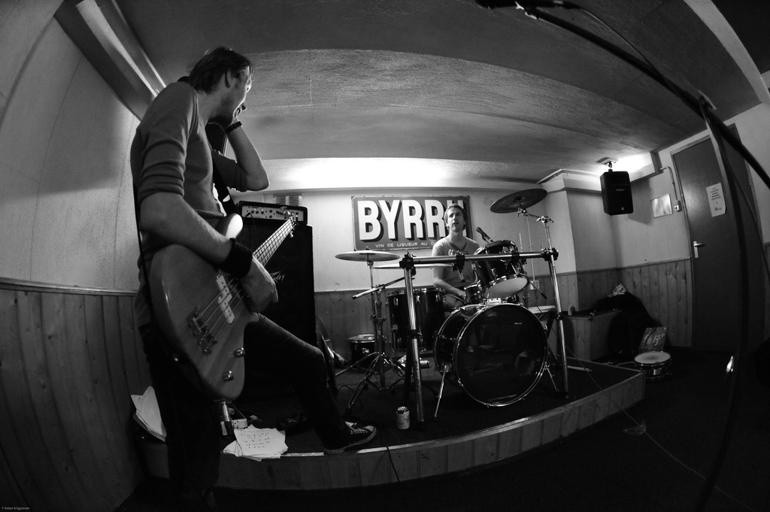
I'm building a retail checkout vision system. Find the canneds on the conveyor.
[420,361,430,368]
[396,406,411,432]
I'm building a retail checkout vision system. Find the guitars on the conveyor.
[148,216,297,400]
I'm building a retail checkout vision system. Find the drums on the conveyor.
[463,284,482,304]
[634,351,672,379]
[470,239,528,298]
[433,301,557,418]
[388,288,445,350]
[346,334,385,368]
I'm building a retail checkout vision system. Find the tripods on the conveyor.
[332,296,413,380]
[344,337,441,414]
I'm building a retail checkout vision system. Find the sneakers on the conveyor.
[325,424,377,455]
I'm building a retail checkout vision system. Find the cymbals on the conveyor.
[490,189,547,213]
[335,249,400,261]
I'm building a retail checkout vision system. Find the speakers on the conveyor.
[238,216,317,347]
[599,170,634,216]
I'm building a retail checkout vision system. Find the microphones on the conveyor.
[475,225,493,242]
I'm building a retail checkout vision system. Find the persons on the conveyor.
[130,44,377,512]
[430,204,480,309]
